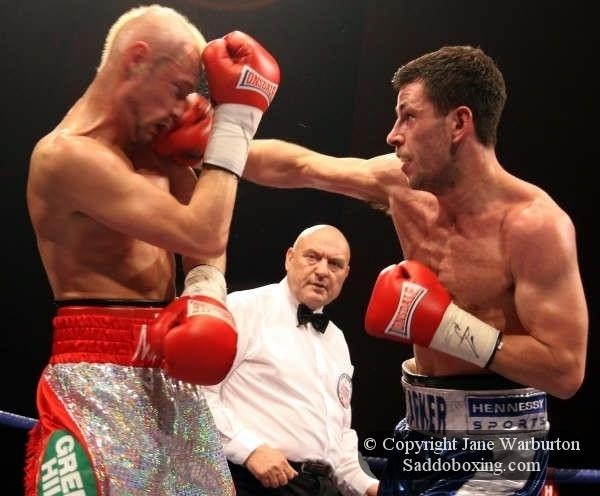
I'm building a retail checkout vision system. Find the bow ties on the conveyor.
[297,302,330,333]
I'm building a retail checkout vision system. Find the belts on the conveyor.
[289,461,331,476]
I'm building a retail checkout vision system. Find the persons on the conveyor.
[197,225,383,496]
[26,5,282,496]
[149,45,590,496]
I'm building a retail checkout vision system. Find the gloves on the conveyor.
[149,264,238,386]
[364,259,503,370]
[151,92,214,170]
[202,30,280,177]
